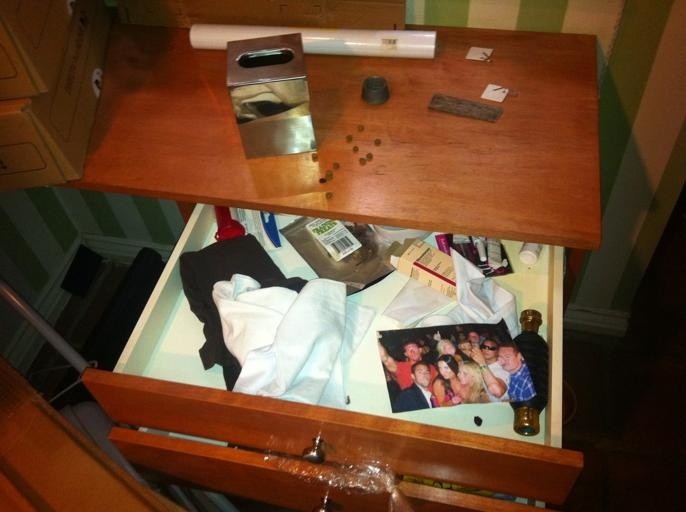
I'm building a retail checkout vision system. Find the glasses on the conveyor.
[481,344,498,351]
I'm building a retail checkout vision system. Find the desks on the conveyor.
[67,26,605,512]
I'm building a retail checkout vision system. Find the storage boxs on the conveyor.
[1,0,113,189]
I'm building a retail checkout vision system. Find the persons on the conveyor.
[379,329,538,414]
